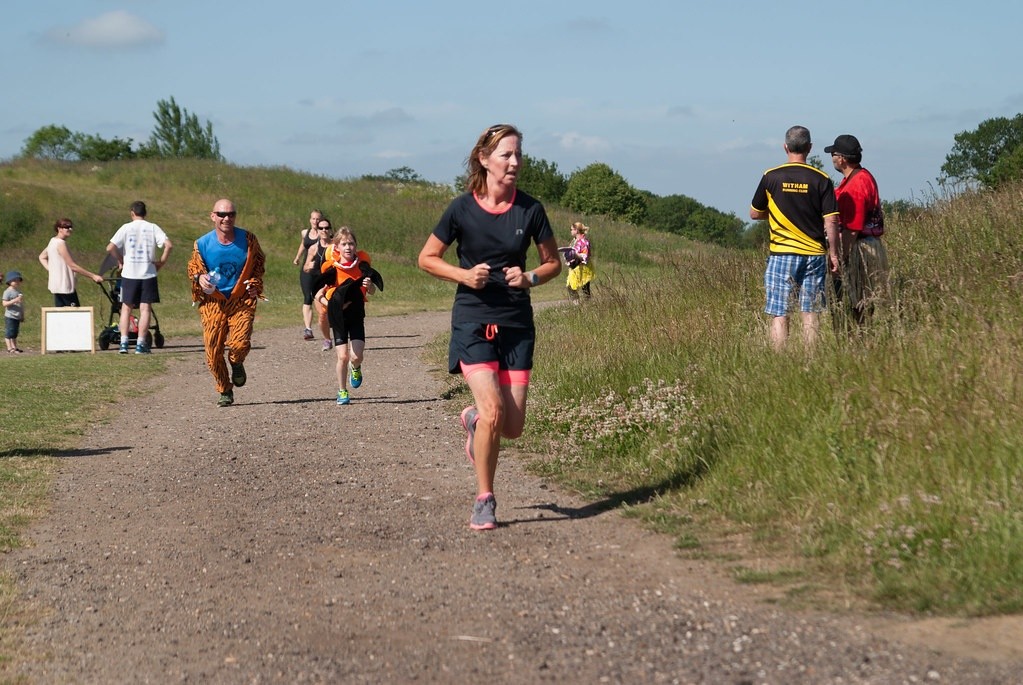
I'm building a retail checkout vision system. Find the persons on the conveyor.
[824,135,889,342]
[2,272,24,353]
[564,222,591,302]
[107,201,172,355]
[189,199,265,407]
[418,125,562,531]
[749,126,839,352]
[314,226,384,405]
[293,209,337,351]
[39,218,103,307]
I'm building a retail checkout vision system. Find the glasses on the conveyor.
[482,124,504,145]
[831,152,840,157]
[212,210,237,218]
[60,225,72,229]
[318,226,330,230]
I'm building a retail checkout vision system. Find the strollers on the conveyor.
[98,248,165,350]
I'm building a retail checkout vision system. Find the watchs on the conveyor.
[193,274,199,282]
[527,270,538,287]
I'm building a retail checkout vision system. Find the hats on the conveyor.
[824,135,863,155]
[5,271,23,286]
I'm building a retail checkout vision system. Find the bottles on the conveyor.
[201,267,221,294]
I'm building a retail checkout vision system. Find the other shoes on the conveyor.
[7,348,24,354]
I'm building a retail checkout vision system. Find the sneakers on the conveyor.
[461,407,479,462]
[217,390,235,407]
[349,363,363,388]
[231,364,247,387]
[470,493,499,530]
[303,329,314,340]
[135,342,151,354]
[119,342,128,354]
[337,389,351,405]
[322,338,333,351]
